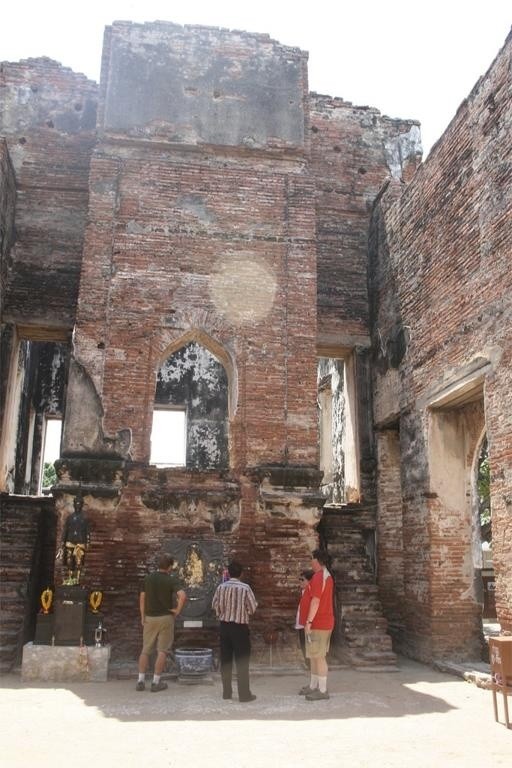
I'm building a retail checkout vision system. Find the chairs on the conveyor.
[489,653,512,730]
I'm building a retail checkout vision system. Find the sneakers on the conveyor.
[299,684,329,700]
[151,683,168,692]
[136,681,145,691]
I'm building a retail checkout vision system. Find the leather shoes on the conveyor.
[240,695,256,702]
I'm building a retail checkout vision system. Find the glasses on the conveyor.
[299,578,304,581]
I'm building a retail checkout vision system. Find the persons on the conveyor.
[295,549,335,700]
[211,562,258,703]
[136,557,186,692]
[61,496,90,585]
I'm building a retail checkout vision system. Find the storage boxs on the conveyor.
[488,636,512,686]
